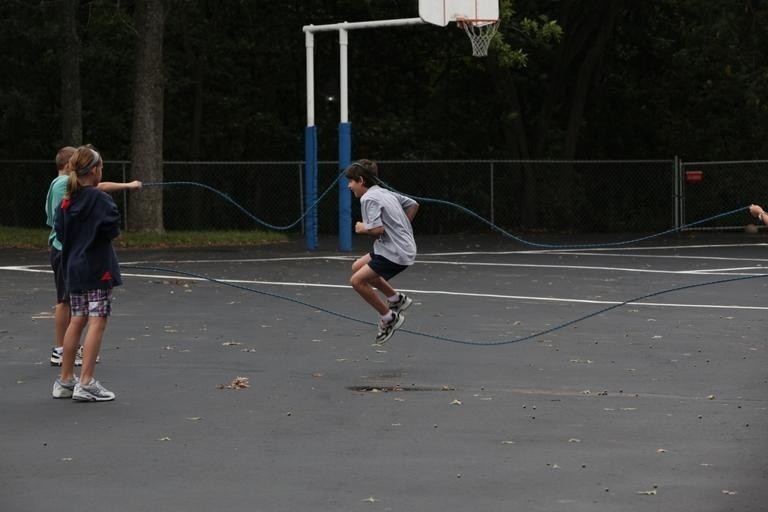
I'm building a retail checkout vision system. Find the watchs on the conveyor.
[758,211,765,222]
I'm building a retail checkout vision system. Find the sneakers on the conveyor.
[375,308,405,345]
[386,292,413,314]
[52,374,80,399]
[72,377,115,402]
[50,344,100,367]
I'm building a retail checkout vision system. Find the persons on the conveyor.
[343,158,421,345]
[52,146,124,402]
[750,203,768,226]
[44,146,144,366]
